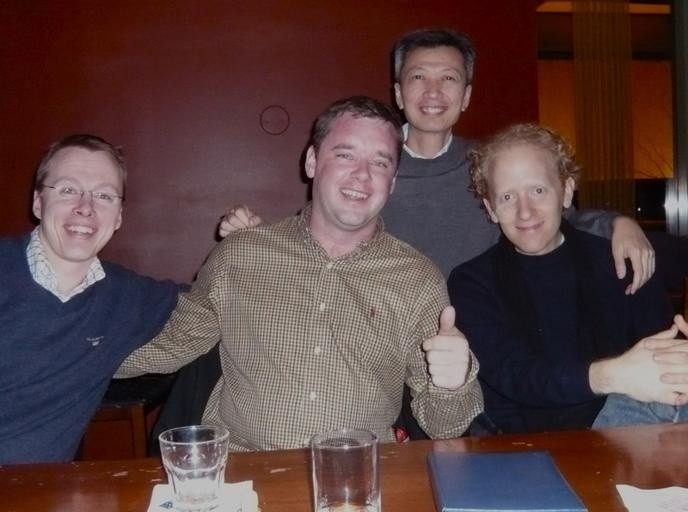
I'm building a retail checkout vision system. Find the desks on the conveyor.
[1,423,687,511]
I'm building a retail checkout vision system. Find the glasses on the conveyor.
[43,184,125,206]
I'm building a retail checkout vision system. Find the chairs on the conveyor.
[75,397,152,461]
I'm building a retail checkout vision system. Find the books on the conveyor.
[426,449,588,512]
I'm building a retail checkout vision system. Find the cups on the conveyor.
[158,424,230,510]
[309,427,383,511]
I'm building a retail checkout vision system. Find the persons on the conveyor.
[113,96,485,455]
[0,134,192,466]
[218,26,656,441]
[447,123,688,437]
